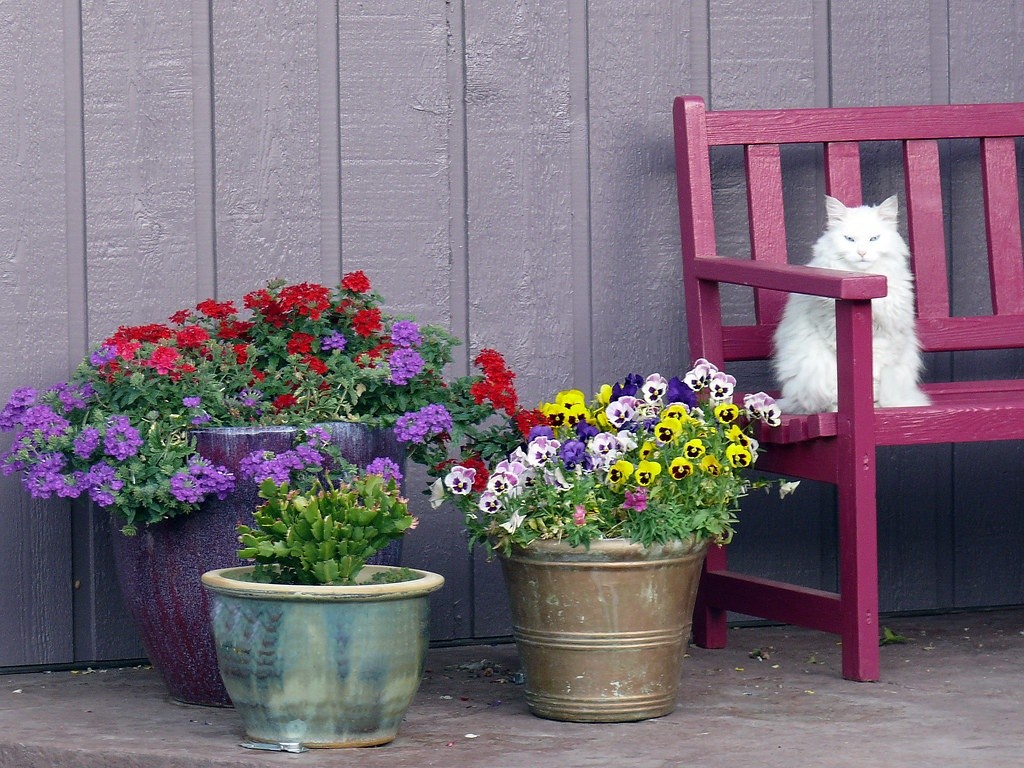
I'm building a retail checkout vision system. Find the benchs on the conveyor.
[672,96,1024,682]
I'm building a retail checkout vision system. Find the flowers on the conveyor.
[0,268,802,563]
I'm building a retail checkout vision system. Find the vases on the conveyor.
[107,424,409,708]
[490,534,718,722]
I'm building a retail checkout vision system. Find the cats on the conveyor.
[768,193,932,416]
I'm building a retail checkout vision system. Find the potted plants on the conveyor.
[199,469,445,749]
